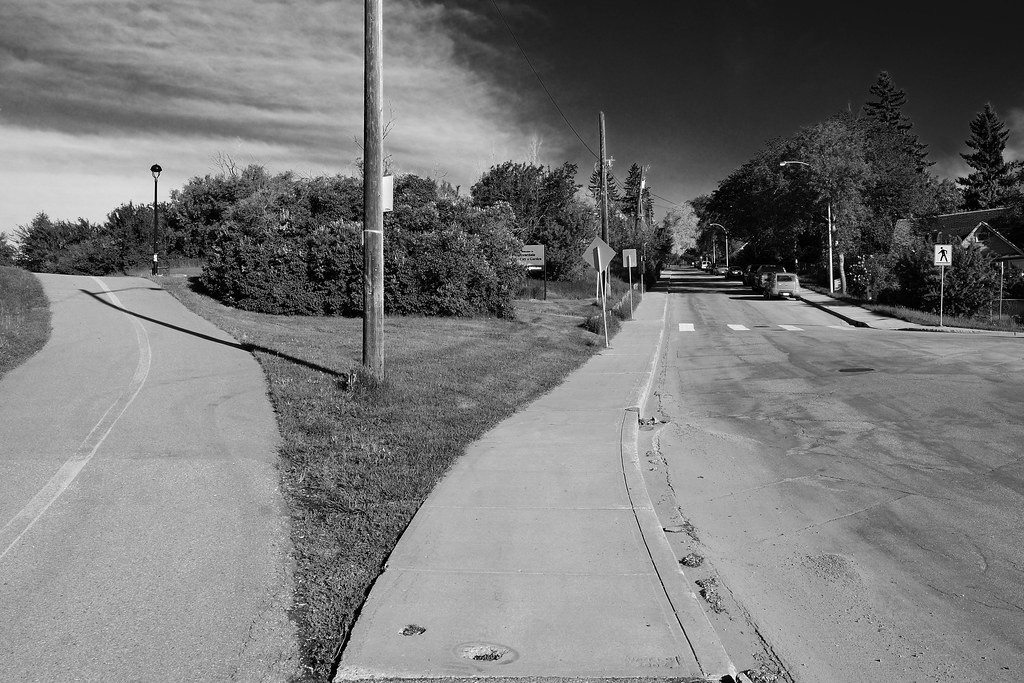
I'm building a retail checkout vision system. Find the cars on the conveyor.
[763,273,801,301]
[693,261,786,294]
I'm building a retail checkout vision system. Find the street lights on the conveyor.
[778,160,834,294]
[709,223,729,268]
[149,163,164,278]
